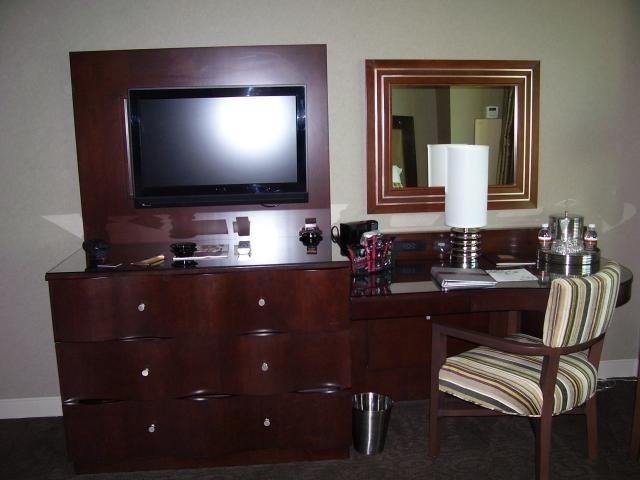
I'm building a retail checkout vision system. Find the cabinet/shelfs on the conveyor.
[41,233,353,474]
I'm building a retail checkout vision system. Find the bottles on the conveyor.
[538,223,551,249]
[538,270,551,288]
[585,224,598,251]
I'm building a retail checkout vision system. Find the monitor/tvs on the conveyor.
[128,84,309,208]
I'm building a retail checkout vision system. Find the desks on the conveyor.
[349,227,634,408]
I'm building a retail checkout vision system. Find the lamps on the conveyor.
[427,143,444,187]
[444,143,490,263]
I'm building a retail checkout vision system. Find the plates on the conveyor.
[168,241,198,257]
[172,259,199,270]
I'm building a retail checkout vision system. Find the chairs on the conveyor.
[425,258,621,479]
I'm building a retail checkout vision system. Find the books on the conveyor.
[430,266,498,288]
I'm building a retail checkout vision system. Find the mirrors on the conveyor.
[364,58,541,215]
